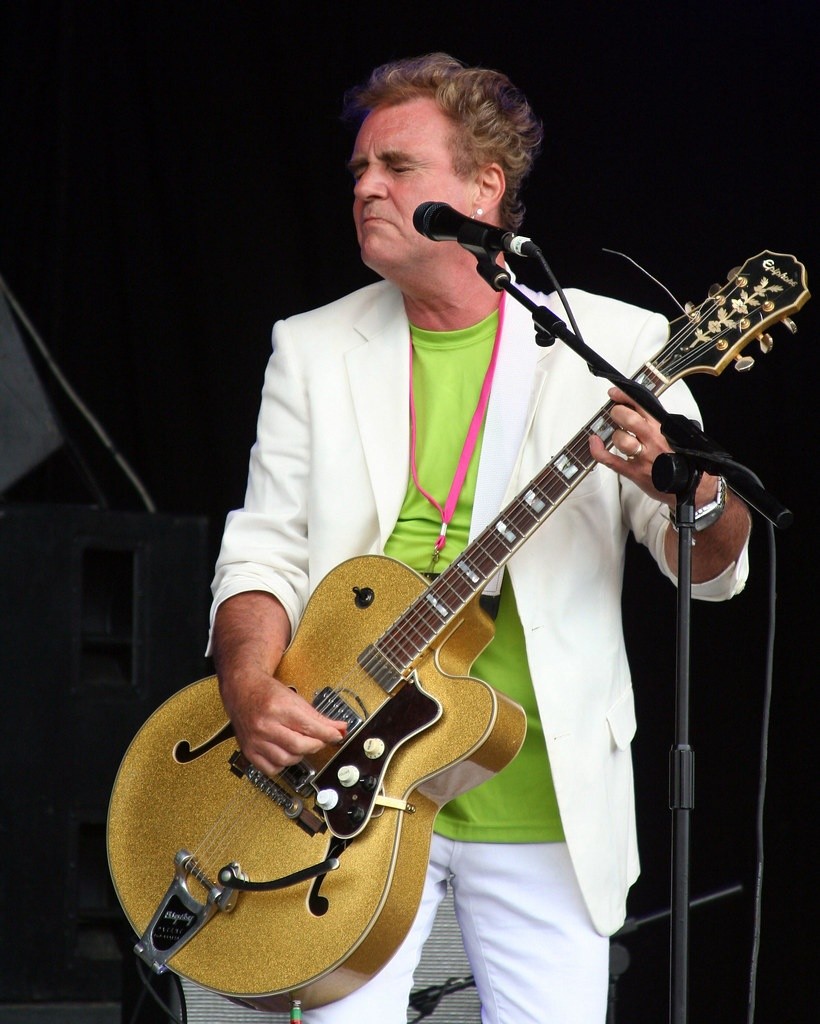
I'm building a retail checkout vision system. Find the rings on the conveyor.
[628,444,644,460]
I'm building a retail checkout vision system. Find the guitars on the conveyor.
[105,251,810,1015]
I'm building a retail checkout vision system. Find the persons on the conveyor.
[204,54,750,1024]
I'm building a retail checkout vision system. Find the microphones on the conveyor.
[413,201,541,260]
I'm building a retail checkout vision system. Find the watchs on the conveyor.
[668,477,728,536]
